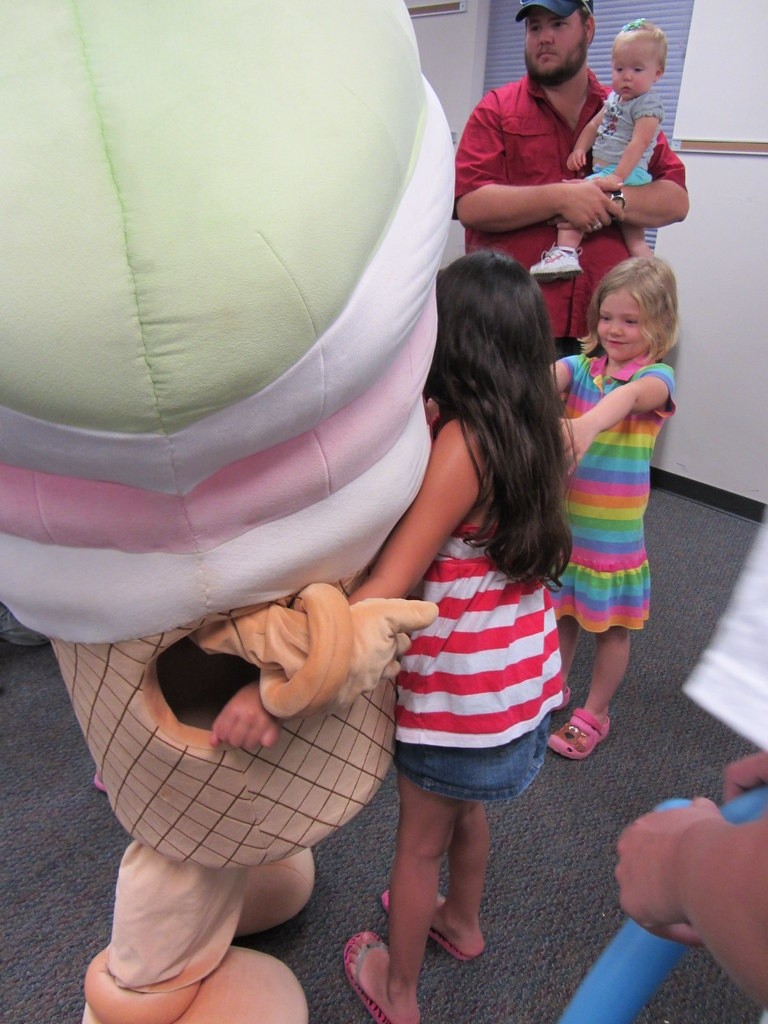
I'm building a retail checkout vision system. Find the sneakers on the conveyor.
[530,244,584,281]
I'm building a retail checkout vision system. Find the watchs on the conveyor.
[610,189,626,220]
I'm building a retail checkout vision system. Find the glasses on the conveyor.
[519,0,593,16]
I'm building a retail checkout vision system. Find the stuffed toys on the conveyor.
[0,0,474,1024]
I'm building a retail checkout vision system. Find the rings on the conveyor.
[594,223,601,229]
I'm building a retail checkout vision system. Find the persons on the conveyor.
[528,19,668,282]
[614,751,768,1012]
[206,251,574,1024]
[547,256,678,758]
[453,0,689,360]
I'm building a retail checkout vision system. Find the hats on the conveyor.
[515,0,593,22]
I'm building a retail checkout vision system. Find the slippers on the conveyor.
[549,686,570,712]
[381,888,472,961]
[342,931,390,1024]
[547,707,609,759]
